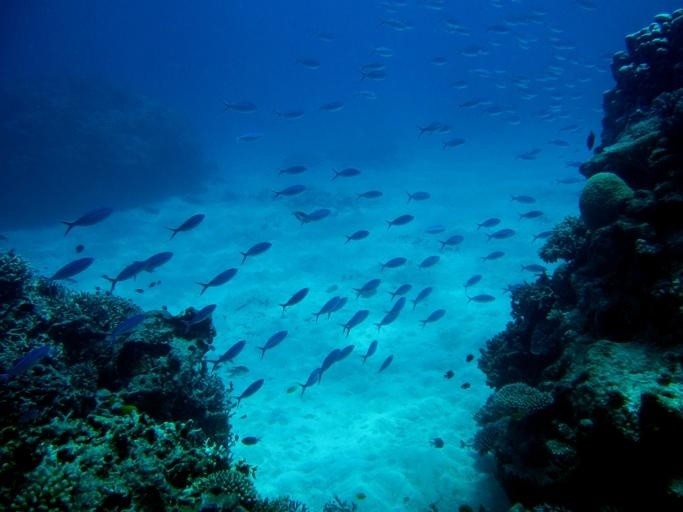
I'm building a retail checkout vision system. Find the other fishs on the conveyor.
[1,166,584,409]
[223,5,623,164]
